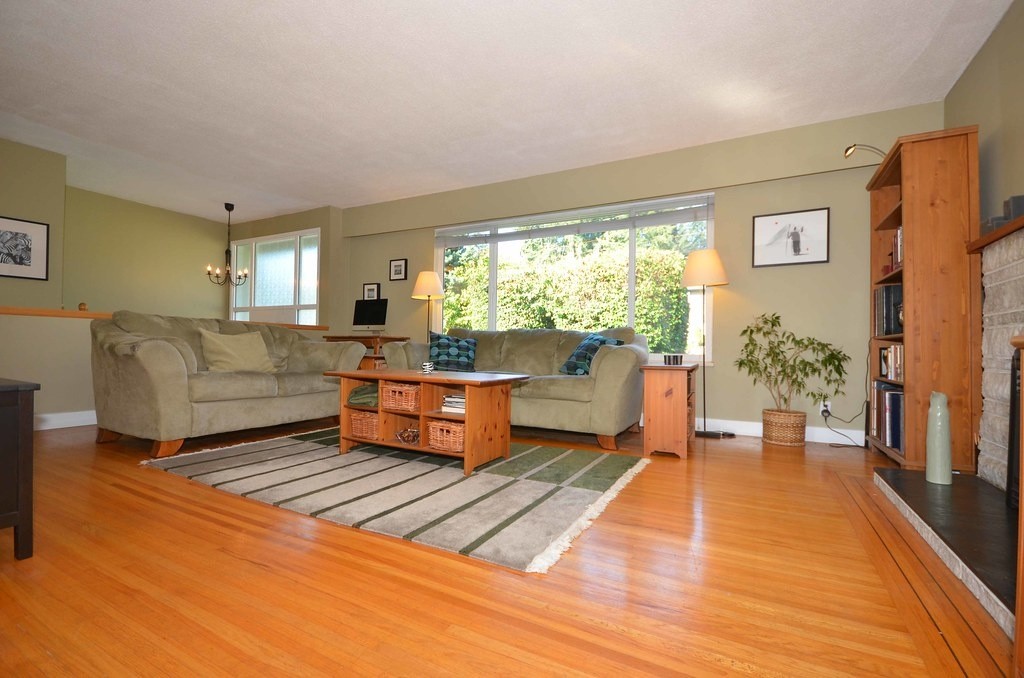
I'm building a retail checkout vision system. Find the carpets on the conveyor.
[138,425,653,576]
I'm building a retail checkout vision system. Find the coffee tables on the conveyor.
[324,371,528,477]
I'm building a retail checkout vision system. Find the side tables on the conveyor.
[640,362,699,459]
[357,355,387,371]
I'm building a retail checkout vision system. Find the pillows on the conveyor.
[197,327,278,373]
[429,330,478,373]
[558,332,624,376]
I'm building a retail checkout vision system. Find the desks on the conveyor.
[0,377,41,559]
[322,335,410,355]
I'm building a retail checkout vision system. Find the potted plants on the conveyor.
[733,312,852,446]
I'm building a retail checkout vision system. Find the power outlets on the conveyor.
[820,401,831,416]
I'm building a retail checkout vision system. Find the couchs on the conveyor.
[89,311,368,457]
[381,328,649,452]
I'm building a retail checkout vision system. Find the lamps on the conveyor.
[206,202,249,287]
[411,270,446,343]
[844,144,887,158]
[680,247,736,437]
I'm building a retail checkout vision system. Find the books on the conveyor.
[442,394,465,414]
[873,225,904,453]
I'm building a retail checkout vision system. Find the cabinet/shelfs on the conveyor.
[865,123,984,473]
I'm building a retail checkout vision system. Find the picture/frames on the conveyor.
[0,216,49,281]
[389,258,408,281]
[752,207,830,268]
[363,282,380,300]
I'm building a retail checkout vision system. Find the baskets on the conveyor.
[381,382,421,412]
[351,412,379,439]
[427,420,465,453]
